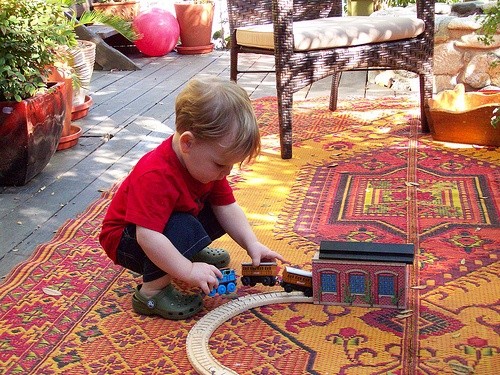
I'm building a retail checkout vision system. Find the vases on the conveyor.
[174,2,215,55]
[92,1,136,22]
[67,40,96,106]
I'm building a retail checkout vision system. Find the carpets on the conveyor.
[0,96,500,375]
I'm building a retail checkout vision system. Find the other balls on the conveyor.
[134,8,180,57]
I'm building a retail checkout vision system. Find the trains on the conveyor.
[204,260,314,299]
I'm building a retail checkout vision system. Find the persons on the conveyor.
[98,76,286,320]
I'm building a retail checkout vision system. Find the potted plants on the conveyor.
[39,0,143,137]
[0,0,79,187]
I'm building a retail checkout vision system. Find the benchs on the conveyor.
[227,0,435,160]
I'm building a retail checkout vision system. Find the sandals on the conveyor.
[191,247,230,269]
[131,283,203,320]
